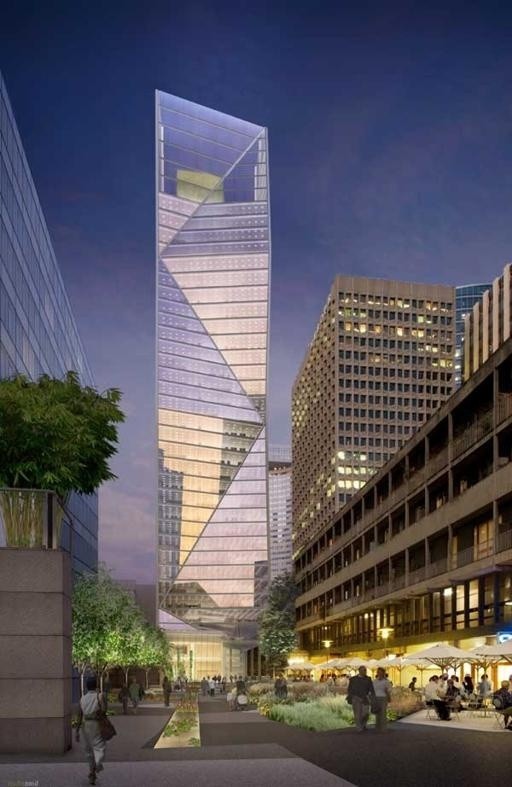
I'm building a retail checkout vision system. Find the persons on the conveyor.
[375,673,394,687]
[408,677,422,701]
[347,665,376,733]
[372,667,392,734]
[162,669,351,712]
[74,676,108,787]
[104,676,148,716]
[422,673,512,731]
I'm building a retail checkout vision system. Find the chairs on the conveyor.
[424,695,512,729]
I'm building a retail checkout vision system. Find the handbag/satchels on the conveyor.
[96,708,116,742]
[346,695,352,705]
[385,688,391,702]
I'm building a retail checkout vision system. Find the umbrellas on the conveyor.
[283,638,512,673]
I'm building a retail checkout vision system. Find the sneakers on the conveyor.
[95,763,105,773]
[90,776,98,786]
